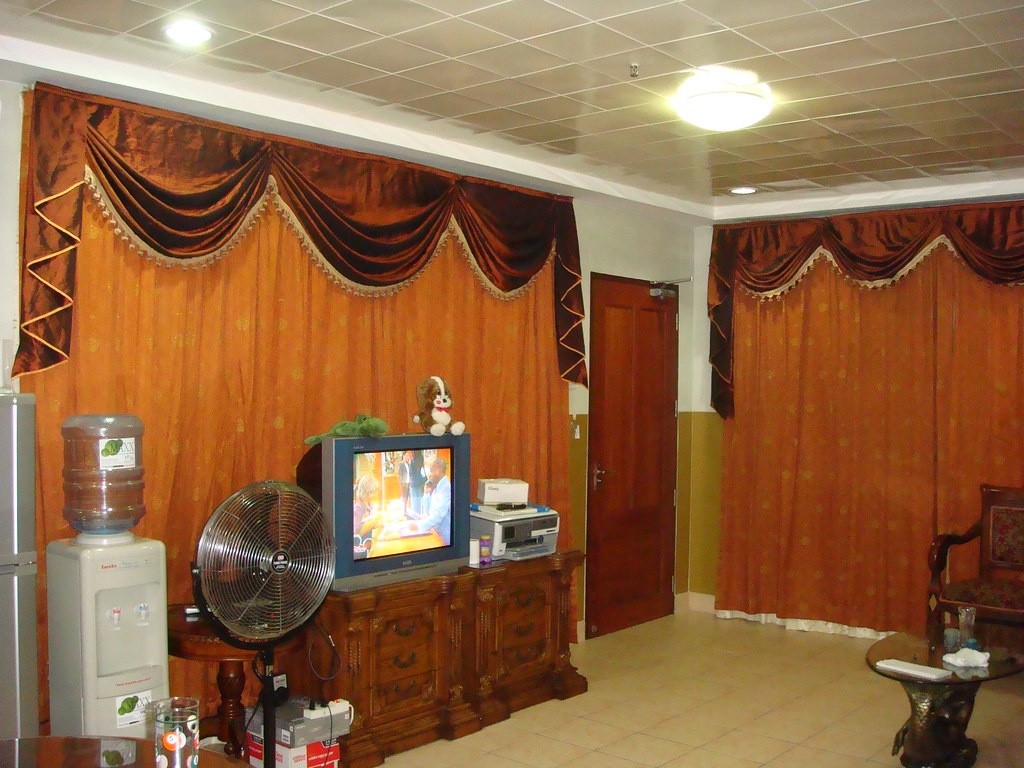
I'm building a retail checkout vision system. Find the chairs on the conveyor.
[928,483,1024,625]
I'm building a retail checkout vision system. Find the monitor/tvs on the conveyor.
[296,432,470,593]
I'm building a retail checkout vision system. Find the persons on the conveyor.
[398,450,452,547]
[353,477,383,555]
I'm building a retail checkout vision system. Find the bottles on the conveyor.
[61,415,145,535]
[479,535,491,563]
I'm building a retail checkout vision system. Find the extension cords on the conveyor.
[303,698,350,720]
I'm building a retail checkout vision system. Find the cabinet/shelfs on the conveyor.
[274,549,587,768]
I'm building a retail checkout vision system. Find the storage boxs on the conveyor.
[245,698,350,768]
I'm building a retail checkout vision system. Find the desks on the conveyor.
[865,624,1024,768]
[168,603,306,753]
[0,736,254,768]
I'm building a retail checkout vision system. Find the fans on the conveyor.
[190,477,338,768]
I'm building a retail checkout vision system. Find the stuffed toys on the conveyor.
[413,374,466,438]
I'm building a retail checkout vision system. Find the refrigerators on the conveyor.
[0,392,39,738]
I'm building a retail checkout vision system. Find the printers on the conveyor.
[469,504,560,561]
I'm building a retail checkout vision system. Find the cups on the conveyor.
[469,539,479,565]
[155,696,200,768]
[944,629,960,648]
[958,606,976,629]
